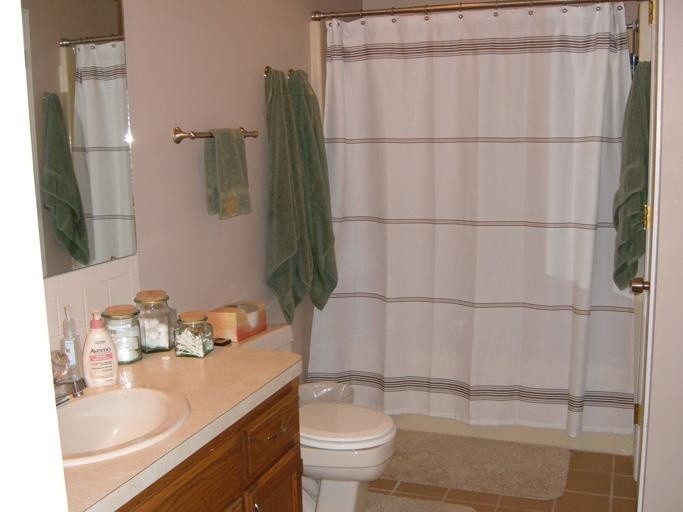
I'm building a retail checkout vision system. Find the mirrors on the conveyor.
[21,0,137,279]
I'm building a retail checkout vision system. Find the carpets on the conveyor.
[379,427,574,503]
[361,489,476,512]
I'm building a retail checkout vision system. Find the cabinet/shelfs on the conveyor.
[115,375,304,512]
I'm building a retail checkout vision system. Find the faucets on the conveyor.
[55,382,83,406]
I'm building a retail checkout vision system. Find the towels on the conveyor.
[609,61,651,292]
[39,90,91,267]
[262,66,340,325]
[203,127,253,220]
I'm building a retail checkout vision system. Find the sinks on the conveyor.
[58,386,192,462]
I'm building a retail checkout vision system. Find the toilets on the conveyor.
[298,401,397,512]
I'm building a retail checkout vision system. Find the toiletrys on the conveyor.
[59,304,82,377]
